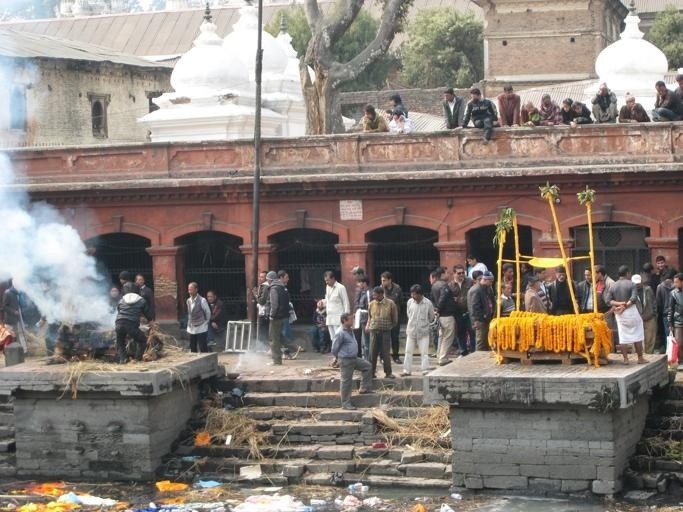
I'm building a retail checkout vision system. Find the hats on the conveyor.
[631,274,642,284]
[349,267,364,273]
[266,271,279,281]
[483,270,494,280]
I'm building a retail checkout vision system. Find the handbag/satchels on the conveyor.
[667,330,679,366]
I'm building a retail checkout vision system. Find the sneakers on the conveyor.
[330,358,453,411]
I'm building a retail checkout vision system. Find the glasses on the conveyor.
[455,271,466,275]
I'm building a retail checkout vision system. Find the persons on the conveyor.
[252,269,300,366]
[430,265,457,365]
[442,87,465,129]
[316,271,350,367]
[329,313,375,410]
[493,84,521,129]
[206,291,226,352]
[399,285,435,375]
[186,282,212,352]
[447,254,494,356]
[606,266,649,365]
[455,88,499,145]
[495,262,618,353]
[519,74,682,130]
[351,267,402,379]
[0,271,154,365]
[631,255,683,364]
[362,93,415,136]
